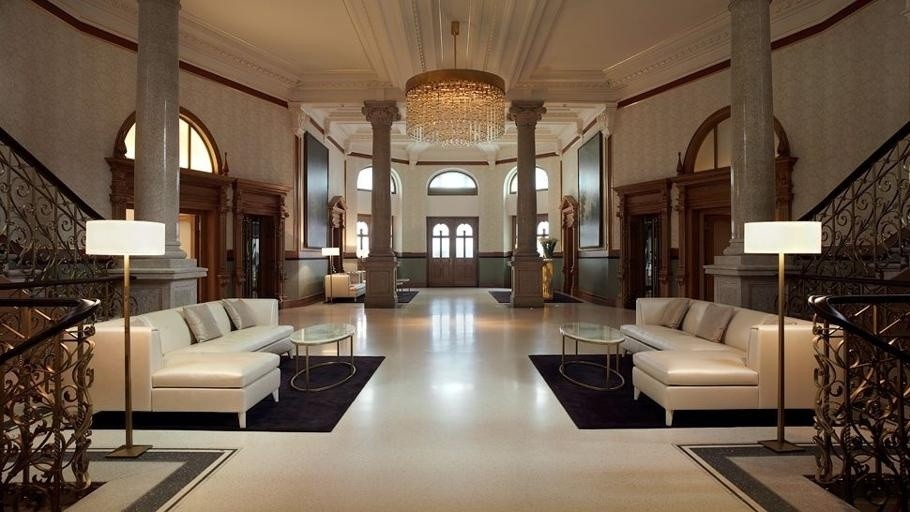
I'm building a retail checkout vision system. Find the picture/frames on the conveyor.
[304,129,331,249]
[573,129,605,250]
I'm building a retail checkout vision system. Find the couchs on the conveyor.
[58,287,297,432]
[325,270,366,302]
[616,291,849,428]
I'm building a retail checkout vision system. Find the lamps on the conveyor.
[320,246,341,306]
[738,219,826,457]
[399,18,508,151]
[81,217,172,461]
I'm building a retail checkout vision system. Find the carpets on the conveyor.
[488,291,585,304]
[322,288,419,303]
[62,350,386,437]
[526,345,845,432]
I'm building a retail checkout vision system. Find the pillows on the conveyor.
[658,296,692,331]
[346,271,364,286]
[691,302,738,345]
[216,295,257,333]
[182,297,224,345]
